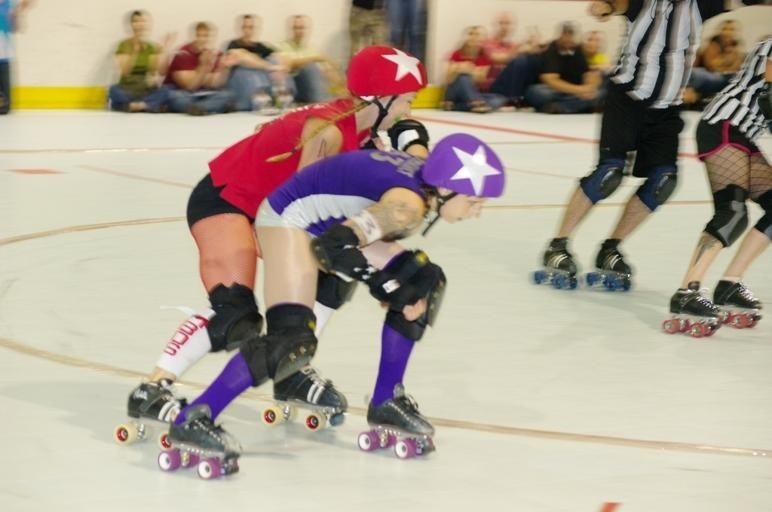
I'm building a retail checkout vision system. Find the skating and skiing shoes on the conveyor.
[158,421,241,480]
[530,239,580,290]
[264,374,347,432]
[111,383,187,445]
[587,240,633,292]
[359,401,437,460]
[663,287,720,338]
[713,275,763,331]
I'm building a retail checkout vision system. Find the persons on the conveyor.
[158,118,506,474]
[441,11,610,115]
[108,2,427,117]
[0,0,29,116]
[682,19,745,105]
[534,1,772,292]
[117,43,429,448]
[664,38,771,335]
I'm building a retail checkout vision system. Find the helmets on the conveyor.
[422,132,507,198]
[348,44,428,97]
